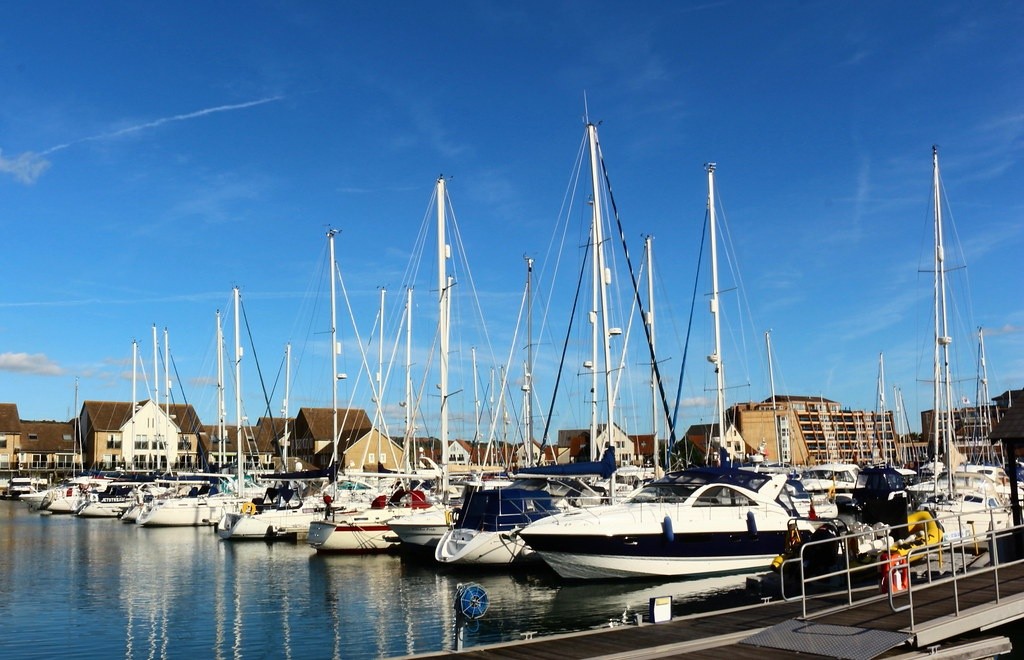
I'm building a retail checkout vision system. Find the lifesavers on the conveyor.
[445,511,452,524]
[882,553,909,592]
[241,503,256,515]
[828,486,835,498]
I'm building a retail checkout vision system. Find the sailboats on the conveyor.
[0,92,1023,580]
